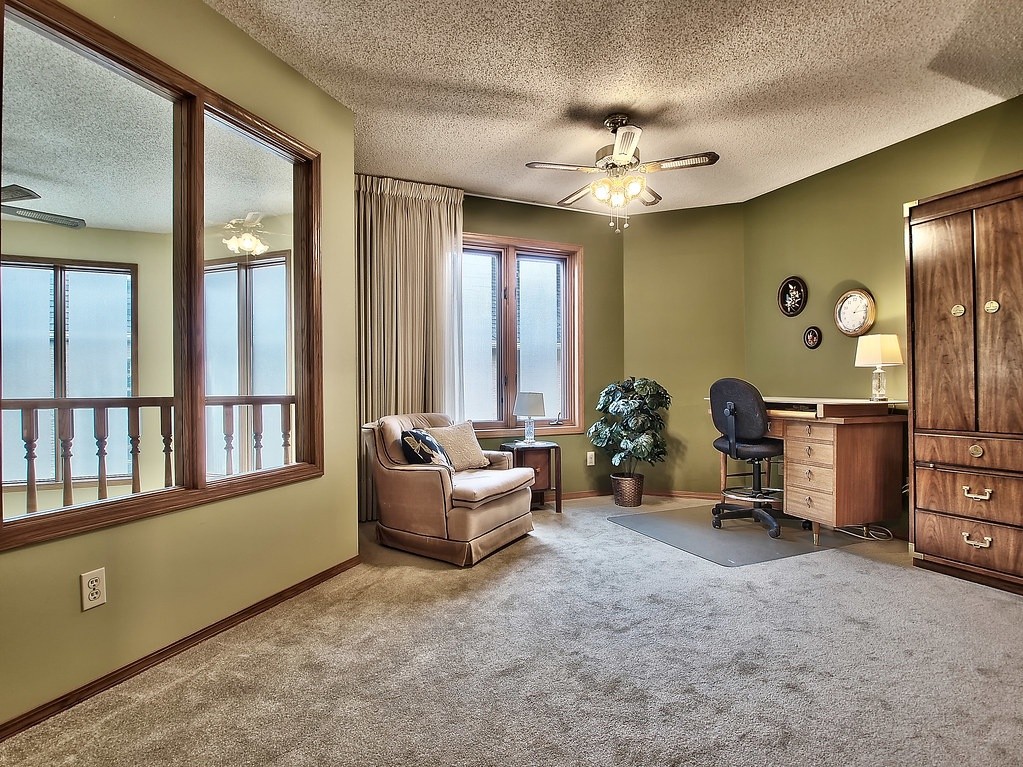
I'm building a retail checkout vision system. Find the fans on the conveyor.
[0,170,86,239]
[507,112,726,215]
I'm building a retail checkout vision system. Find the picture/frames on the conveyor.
[776,274,808,317]
[803,327,822,350]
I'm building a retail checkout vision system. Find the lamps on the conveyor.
[580,168,650,233]
[222,228,269,256]
[513,386,546,442]
[846,335,908,404]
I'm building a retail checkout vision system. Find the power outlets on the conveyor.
[82,562,108,614]
[587,452,595,466]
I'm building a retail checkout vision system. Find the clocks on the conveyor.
[831,289,878,337]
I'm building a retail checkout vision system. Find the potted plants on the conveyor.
[582,377,676,507]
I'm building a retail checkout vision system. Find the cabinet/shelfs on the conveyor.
[905,171,1023,596]
[703,397,911,546]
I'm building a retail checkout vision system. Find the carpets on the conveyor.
[609,497,872,568]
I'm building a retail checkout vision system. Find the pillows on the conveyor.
[402,430,451,467]
[425,420,491,471]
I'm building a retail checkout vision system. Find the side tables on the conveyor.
[500,441,561,516]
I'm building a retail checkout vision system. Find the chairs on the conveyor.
[706,379,812,539]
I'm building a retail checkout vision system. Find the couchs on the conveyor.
[363,411,537,560]
